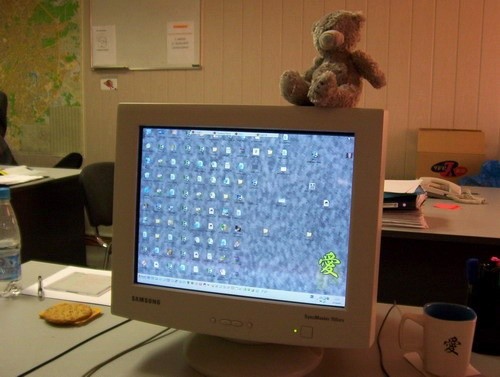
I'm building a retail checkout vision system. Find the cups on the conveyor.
[398,302,477,377]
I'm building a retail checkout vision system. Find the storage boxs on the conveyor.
[417,127,487,183]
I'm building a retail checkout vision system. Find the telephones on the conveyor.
[418,176,461,201]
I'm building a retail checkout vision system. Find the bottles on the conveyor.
[0,188,22,299]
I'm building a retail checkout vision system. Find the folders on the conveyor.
[382,178,427,213]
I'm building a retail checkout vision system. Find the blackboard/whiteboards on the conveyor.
[90,0,202,71]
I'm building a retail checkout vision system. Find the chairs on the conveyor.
[78,162,115,270]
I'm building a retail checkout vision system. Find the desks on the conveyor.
[0,165,86,268]
[0,260,500,377]
[381,187,500,246]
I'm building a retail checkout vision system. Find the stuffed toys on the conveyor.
[279,9,388,108]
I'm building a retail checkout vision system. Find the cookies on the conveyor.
[43,303,92,323]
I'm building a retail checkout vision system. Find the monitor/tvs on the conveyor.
[111,102,387,377]
